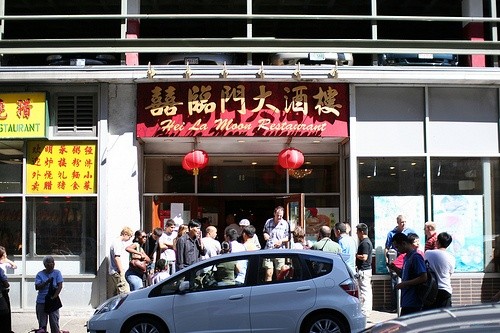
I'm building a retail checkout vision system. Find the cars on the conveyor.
[360,300,500,333]
[19,236,97,270]
[86,249,366,333]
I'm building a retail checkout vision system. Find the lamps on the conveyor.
[288,161,313,179]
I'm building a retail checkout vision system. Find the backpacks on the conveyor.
[129,249,151,276]
[409,253,439,307]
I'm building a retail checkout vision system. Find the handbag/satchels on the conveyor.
[200,271,218,289]
[44,279,62,314]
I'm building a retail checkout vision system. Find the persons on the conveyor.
[424,221,439,250]
[34,256,64,333]
[108,217,221,295]
[224,229,247,286]
[0,246,17,333]
[238,218,261,251]
[310,225,342,274]
[262,206,289,283]
[390,233,425,279]
[345,223,351,235]
[383,214,417,314]
[424,232,456,310]
[388,233,427,316]
[290,227,311,280]
[355,223,373,310]
[334,223,357,278]
[242,225,259,250]
[211,241,239,287]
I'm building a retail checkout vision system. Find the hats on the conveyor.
[239,219,250,227]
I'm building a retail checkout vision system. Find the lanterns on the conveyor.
[182,139,208,175]
[278,140,305,174]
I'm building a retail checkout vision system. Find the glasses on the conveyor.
[142,236,147,238]
